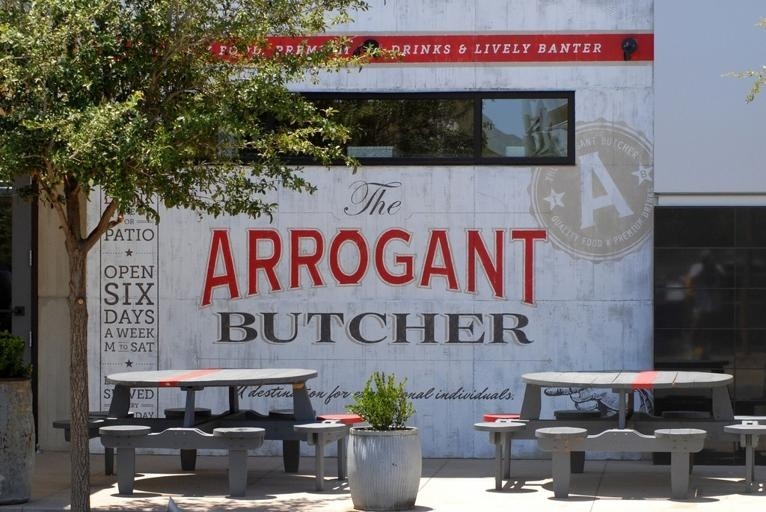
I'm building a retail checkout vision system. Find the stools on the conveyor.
[473,410,766,500]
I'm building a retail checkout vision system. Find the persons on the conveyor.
[682,251,726,362]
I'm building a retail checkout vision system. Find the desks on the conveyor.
[519,369,735,421]
[105,368,318,422]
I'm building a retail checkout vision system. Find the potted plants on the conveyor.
[0,330,37,505]
[345,371,423,511]
